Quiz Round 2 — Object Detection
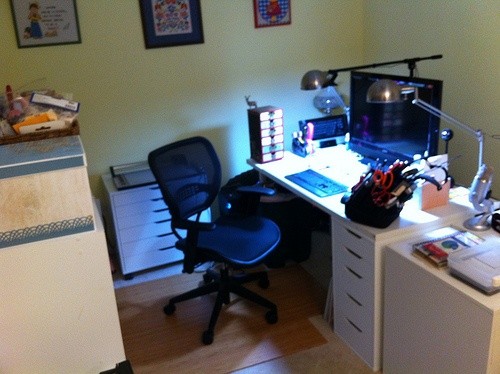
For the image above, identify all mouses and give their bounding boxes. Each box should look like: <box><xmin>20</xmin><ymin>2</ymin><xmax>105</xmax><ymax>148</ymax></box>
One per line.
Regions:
<box><xmin>340</xmin><ymin>192</ymin><xmax>353</xmax><ymax>204</ymax></box>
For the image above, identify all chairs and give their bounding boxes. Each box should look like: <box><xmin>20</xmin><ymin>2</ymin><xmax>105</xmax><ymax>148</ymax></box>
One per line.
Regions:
<box><xmin>150</xmin><ymin>136</ymin><xmax>283</xmax><ymax>345</ymax></box>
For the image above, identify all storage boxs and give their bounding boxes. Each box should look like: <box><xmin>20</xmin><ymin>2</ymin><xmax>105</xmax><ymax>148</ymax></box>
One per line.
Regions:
<box><xmin>0</xmin><ymin>134</ymin><xmax>96</xmax><ymax>249</ymax></box>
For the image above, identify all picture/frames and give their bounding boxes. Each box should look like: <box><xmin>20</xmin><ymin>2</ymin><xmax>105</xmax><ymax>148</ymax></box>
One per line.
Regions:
<box><xmin>253</xmin><ymin>0</ymin><xmax>292</xmax><ymax>28</ymax></box>
<box><xmin>138</xmin><ymin>0</ymin><xmax>204</xmax><ymax>49</ymax></box>
<box><xmin>9</xmin><ymin>0</ymin><xmax>84</xmax><ymax>49</ymax></box>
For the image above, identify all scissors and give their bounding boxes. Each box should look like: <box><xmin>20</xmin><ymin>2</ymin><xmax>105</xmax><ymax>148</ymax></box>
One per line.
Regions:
<box><xmin>373</xmin><ymin>170</ymin><xmax>394</xmax><ymax>205</ymax></box>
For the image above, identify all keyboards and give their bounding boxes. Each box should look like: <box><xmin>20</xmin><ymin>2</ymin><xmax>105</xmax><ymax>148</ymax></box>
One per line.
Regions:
<box><xmin>285</xmin><ymin>169</ymin><xmax>349</xmax><ymax>197</ymax></box>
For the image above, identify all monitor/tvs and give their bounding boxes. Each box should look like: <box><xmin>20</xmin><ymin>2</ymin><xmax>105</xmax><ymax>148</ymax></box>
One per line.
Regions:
<box><xmin>350</xmin><ymin>70</ymin><xmax>444</xmax><ymax>172</ymax></box>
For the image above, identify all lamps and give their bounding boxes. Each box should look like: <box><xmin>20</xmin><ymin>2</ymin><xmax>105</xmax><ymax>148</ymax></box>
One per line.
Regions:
<box><xmin>366</xmin><ymin>80</ymin><xmax>498</xmax><ymax>231</ymax></box>
<box><xmin>300</xmin><ymin>54</ymin><xmax>443</xmax><ymax>91</ymax></box>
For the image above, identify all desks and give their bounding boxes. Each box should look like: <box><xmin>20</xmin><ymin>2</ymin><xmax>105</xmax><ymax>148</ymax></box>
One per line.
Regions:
<box><xmin>0</xmin><ymin>196</ymin><xmax>128</xmax><ymax>374</ymax></box>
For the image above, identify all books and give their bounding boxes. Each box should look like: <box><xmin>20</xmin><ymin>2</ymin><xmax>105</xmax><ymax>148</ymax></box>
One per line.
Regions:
<box><xmin>413</xmin><ymin>231</ymin><xmax>489</xmax><ymax>268</ymax></box>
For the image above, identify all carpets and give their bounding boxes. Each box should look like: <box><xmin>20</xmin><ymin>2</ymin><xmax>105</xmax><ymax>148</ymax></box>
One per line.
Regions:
<box><xmin>232</xmin><ymin>314</ymin><xmax>374</xmax><ymax>374</ymax></box>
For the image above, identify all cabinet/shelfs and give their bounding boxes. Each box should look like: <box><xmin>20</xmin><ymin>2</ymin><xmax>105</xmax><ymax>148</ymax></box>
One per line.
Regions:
<box><xmin>102</xmin><ymin>176</ymin><xmax>212</xmax><ymax>281</ymax></box>
<box><xmin>246</xmin><ymin>144</ymin><xmax>500</xmax><ymax>374</ymax></box>
